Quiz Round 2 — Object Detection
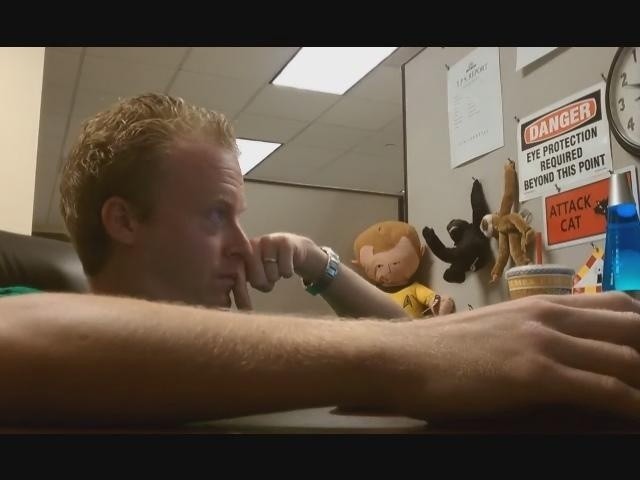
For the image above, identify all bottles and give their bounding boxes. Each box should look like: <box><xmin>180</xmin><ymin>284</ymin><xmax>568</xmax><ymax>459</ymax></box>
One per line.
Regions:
<box><xmin>601</xmin><ymin>172</ymin><xmax>640</xmax><ymax>292</ymax></box>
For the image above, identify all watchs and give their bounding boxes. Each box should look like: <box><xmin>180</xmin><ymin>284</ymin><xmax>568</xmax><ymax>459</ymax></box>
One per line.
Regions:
<box><xmin>301</xmin><ymin>246</ymin><xmax>340</xmax><ymax>296</ymax></box>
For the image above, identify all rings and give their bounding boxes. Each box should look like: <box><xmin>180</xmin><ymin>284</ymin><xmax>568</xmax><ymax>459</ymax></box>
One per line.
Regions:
<box><xmin>263</xmin><ymin>258</ymin><xmax>277</xmax><ymax>263</ymax></box>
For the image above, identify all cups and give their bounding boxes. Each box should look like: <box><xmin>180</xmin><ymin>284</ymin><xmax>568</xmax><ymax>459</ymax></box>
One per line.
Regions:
<box><xmin>505</xmin><ymin>266</ymin><xmax>574</xmax><ymax>300</ymax></box>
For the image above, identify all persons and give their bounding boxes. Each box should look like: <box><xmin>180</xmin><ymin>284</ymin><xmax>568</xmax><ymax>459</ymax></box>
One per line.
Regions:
<box><xmin>0</xmin><ymin>93</ymin><xmax>640</xmax><ymax>429</ymax></box>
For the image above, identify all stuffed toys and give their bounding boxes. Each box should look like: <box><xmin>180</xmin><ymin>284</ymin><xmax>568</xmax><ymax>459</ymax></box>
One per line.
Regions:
<box><xmin>423</xmin><ymin>179</ymin><xmax>489</xmax><ymax>283</ymax></box>
<box><xmin>351</xmin><ymin>220</ymin><xmax>454</xmax><ymax>320</ymax></box>
<box><xmin>480</xmin><ymin>161</ymin><xmax>534</xmax><ymax>286</ymax></box>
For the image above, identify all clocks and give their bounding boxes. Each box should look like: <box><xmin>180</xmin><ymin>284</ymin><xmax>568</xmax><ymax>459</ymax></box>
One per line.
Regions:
<box><xmin>605</xmin><ymin>47</ymin><xmax>640</xmax><ymax>158</ymax></box>
